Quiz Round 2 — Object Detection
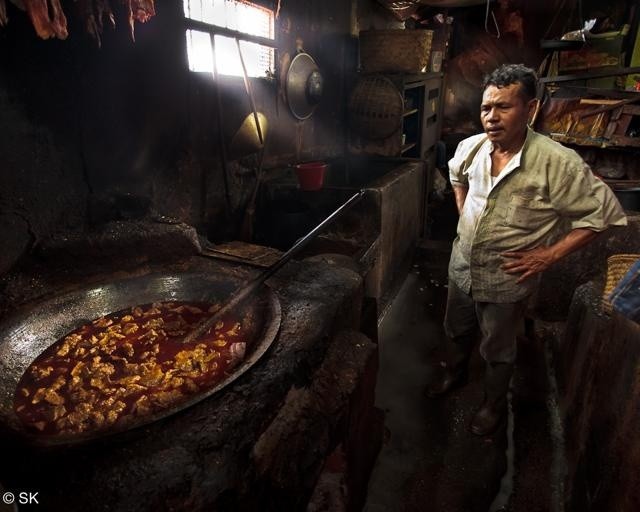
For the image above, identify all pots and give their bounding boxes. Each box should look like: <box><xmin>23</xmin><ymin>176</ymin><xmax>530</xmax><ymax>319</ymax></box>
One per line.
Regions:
<box><xmin>1</xmin><ymin>270</ymin><xmax>284</xmax><ymax>450</ymax></box>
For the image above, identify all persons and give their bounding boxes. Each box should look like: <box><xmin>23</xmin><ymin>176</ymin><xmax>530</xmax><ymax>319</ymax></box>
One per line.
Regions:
<box><xmin>421</xmin><ymin>65</ymin><xmax>629</xmax><ymax>434</ymax></box>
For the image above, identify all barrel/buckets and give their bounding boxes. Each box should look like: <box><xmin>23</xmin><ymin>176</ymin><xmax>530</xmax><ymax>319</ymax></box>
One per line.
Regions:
<box><xmin>294</xmin><ymin>162</ymin><xmax>327</xmax><ymax>191</ymax></box>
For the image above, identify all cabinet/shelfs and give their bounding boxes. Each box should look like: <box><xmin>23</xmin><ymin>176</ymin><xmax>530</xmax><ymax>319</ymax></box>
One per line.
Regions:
<box><xmin>397</xmin><ymin>72</ymin><xmax>442</xmax><ymax>160</ymax></box>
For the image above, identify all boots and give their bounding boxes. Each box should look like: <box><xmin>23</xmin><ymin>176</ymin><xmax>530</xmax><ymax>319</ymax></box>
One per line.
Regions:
<box><xmin>468</xmin><ymin>360</ymin><xmax>514</xmax><ymax>437</ymax></box>
<box><xmin>425</xmin><ymin>340</ymin><xmax>475</xmax><ymax>400</ymax></box>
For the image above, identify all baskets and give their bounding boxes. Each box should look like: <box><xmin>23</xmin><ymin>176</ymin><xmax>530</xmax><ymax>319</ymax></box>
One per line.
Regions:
<box><xmin>358</xmin><ymin>29</ymin><xmax>433</xmax><ymax>74</ymax></box>
<box><xmin>346</xmin><ymin>76</ymin><xmax>405</xmax><ymax>140</ymax></box>
<box><xmin>601</xmin><ymin>254</ymin><xmax>639</xmax><ymax>317</ymax></box>
